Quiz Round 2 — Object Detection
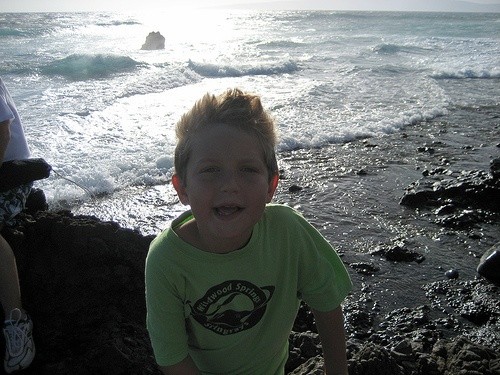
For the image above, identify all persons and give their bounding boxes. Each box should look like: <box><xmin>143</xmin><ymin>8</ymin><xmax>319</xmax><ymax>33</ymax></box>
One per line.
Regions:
<box><xmin>145</xmin><ymin>91</ymin><xmax>353</xmax><ymax>375</ymax></box>
<box><xmin>0</xmin><ymin>78</ymin><xmax>35</xmax><ymax>375</ymax></box>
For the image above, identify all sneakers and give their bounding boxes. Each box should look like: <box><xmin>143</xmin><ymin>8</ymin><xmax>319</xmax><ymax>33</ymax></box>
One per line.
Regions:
<box><xmin>3</xmin><ymin>308</ymin><xmax>38</xmax><ymax>374</ymax></box>
